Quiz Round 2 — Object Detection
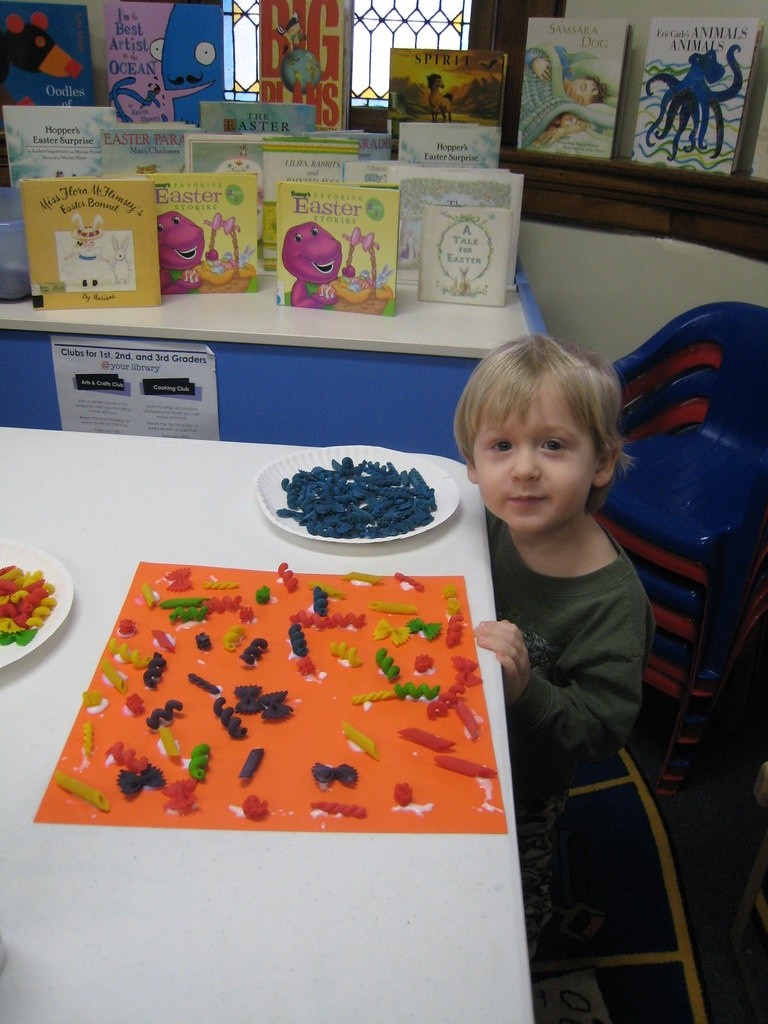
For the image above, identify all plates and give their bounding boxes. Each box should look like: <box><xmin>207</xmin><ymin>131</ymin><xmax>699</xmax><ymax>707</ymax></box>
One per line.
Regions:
<box><xmin>0</xmin><ymin>538</ymin><xmax>75</xmax><ymax>669</ymax></box>
<box><xmin>254</xmin><ymin>443</ymin><xmax>461</xmax><ymax>545</ymax></box>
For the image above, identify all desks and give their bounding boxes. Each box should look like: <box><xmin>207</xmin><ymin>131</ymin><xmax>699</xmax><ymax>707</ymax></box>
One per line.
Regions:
<box><xmin>0</xmin><ymin>427</ymin><xmax>535</xmax><ymax>1024</ymax></box>
<box><xmin>0</xmin><ymin>258</ymin><xmax>548</xmax><ymax>468</ymax></box>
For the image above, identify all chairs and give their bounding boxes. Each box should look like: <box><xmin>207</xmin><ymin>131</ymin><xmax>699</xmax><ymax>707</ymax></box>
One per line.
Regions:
<box><xmin>584</xmin><ymin>303</ymin><xmax>768</xmax><ymax>795</ymax></box>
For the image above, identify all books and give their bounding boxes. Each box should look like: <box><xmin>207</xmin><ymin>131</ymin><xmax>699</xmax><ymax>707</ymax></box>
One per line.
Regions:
<box><xmin>0</xmin><ymin>0</ymin><xmax>524</xmax><ymax>320</ymax></box>
<box><xmin>632</xmin><ymin>16</ymin><xmax>765</xmax><ymax>175</ymax></box>
<box><xmin>750</xmin><ymin>90</ymin><xmax>768</xmax><ymax>183</ymax></box>
<box><xmin>515</xmin><ymin>17</ymin><xmax>635</xmax><ymax>159</ymax></box>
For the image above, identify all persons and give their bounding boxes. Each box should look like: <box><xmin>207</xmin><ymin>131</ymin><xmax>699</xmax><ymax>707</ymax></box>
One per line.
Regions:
<box><xmin>457</xmin><ymin>331</ymin><xmax>654</xmax><ymax>981</ymax></box>
